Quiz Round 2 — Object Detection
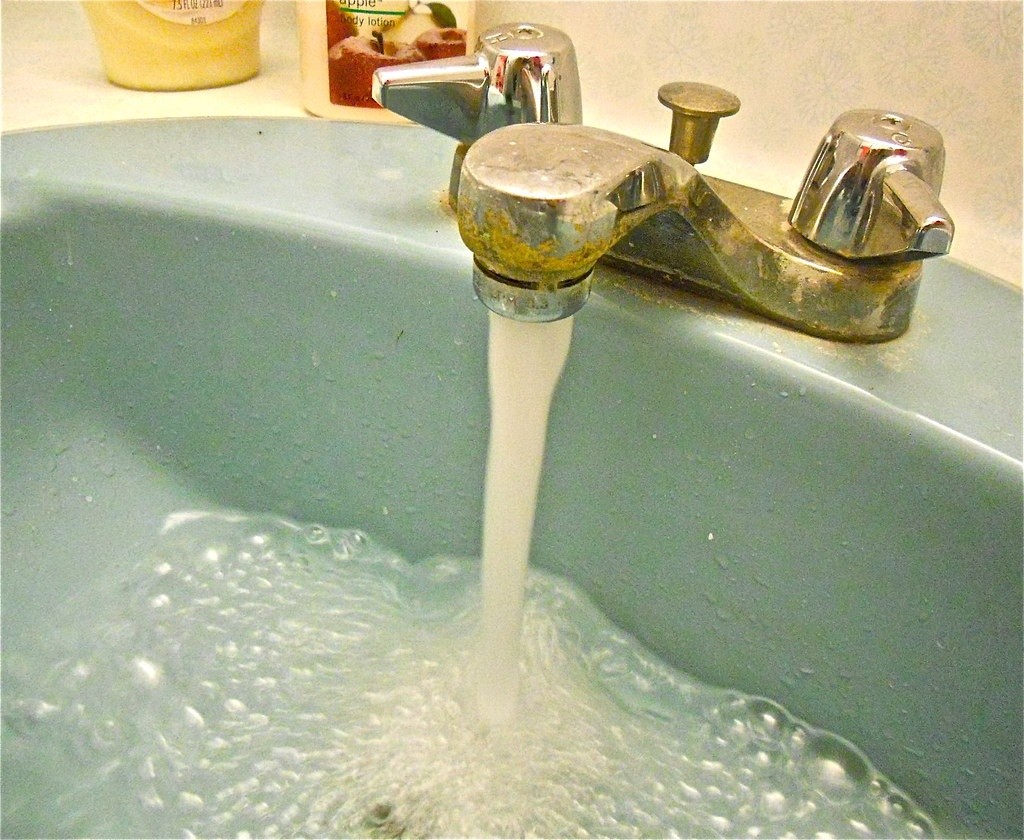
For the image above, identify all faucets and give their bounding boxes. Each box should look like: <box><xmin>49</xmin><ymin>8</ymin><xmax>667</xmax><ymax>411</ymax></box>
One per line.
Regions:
<box><xmin>373</xmin><ymin>23</ymin><xmax>955</xmax><ymax>344</ymax></box>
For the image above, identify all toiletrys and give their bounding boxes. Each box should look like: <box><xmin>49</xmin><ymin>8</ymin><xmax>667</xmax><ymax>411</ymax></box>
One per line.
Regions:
<box><xmin>297</xmin><ymin>0</ymin><xmax>477</xmax><ymax>125</ymax></box>
<box><xmin>82</xmin><ymin>0</ymin><xmax>266</xmax><ymax>92</ymax></box>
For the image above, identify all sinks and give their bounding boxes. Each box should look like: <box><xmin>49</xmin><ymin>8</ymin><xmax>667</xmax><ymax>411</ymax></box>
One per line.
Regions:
<box><xmin>0</xmin><ymin>113</ymin><xmax>1024</xmax><ymax>837</ymax></box>
